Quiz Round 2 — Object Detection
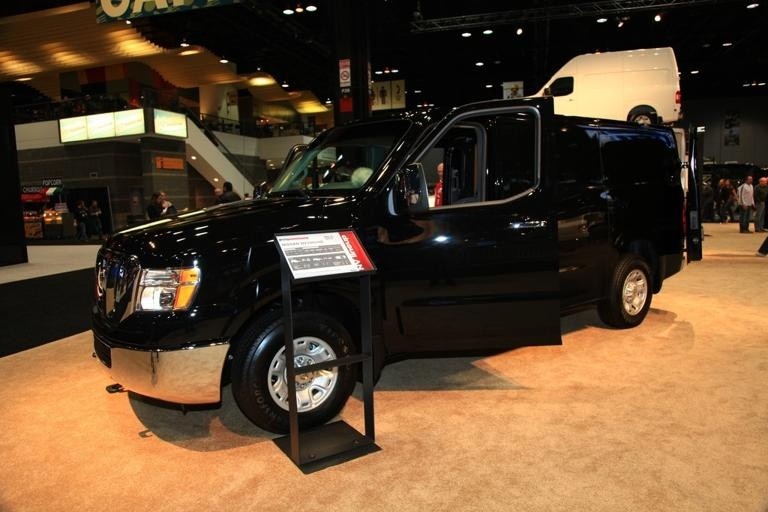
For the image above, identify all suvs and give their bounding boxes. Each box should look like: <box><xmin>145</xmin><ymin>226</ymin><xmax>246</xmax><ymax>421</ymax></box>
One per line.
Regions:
<box><xmin>90</xmin><ymin>96</ymin><xmax>687</xmax><ymax>436</ymax></box>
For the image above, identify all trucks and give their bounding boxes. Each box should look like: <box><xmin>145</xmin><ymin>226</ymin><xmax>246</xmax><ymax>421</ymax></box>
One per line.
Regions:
<box><xmin>525</xmin><ymin>47</ymin><xmax>682</xmax><ymax>125</ymax></box>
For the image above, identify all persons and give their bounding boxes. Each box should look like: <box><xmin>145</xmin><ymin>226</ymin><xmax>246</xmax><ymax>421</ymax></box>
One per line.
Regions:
<box><xmin>756</xmin><ymin>236</ymin><xmax>768</xmax><ymax>255</ymax></box>
<box><xmin>433</xmin><ymin>164</ymin><xmax>444</xmax><ymax>207</ymax></box>
<box><xmin>72</xmin><ymin>201</ymin><xmax>91</xmax><ymax>243</ymax></box>
<box><xmin>703</xmin><ymin>175</ymin><xmax>768</xmax><ymax>235</ymax></box>
<box><xmin>86</xmin><ymin>200</ymin><xmax>107</xmax><ymax>240</ymax></box>
<box><xmin>160</xmin><ymin>192</ymin><xmax>177</xmax><ymax>216</ymax></box>
<box><xmin>216</xmin><ymin>182</ymin><xmax>241</xmax><ymax>205</ymax></box>
<box><xmin>148</xmin><ymin>193</ymin><xmax>169</xmax><ymax>218</ymax></box>
